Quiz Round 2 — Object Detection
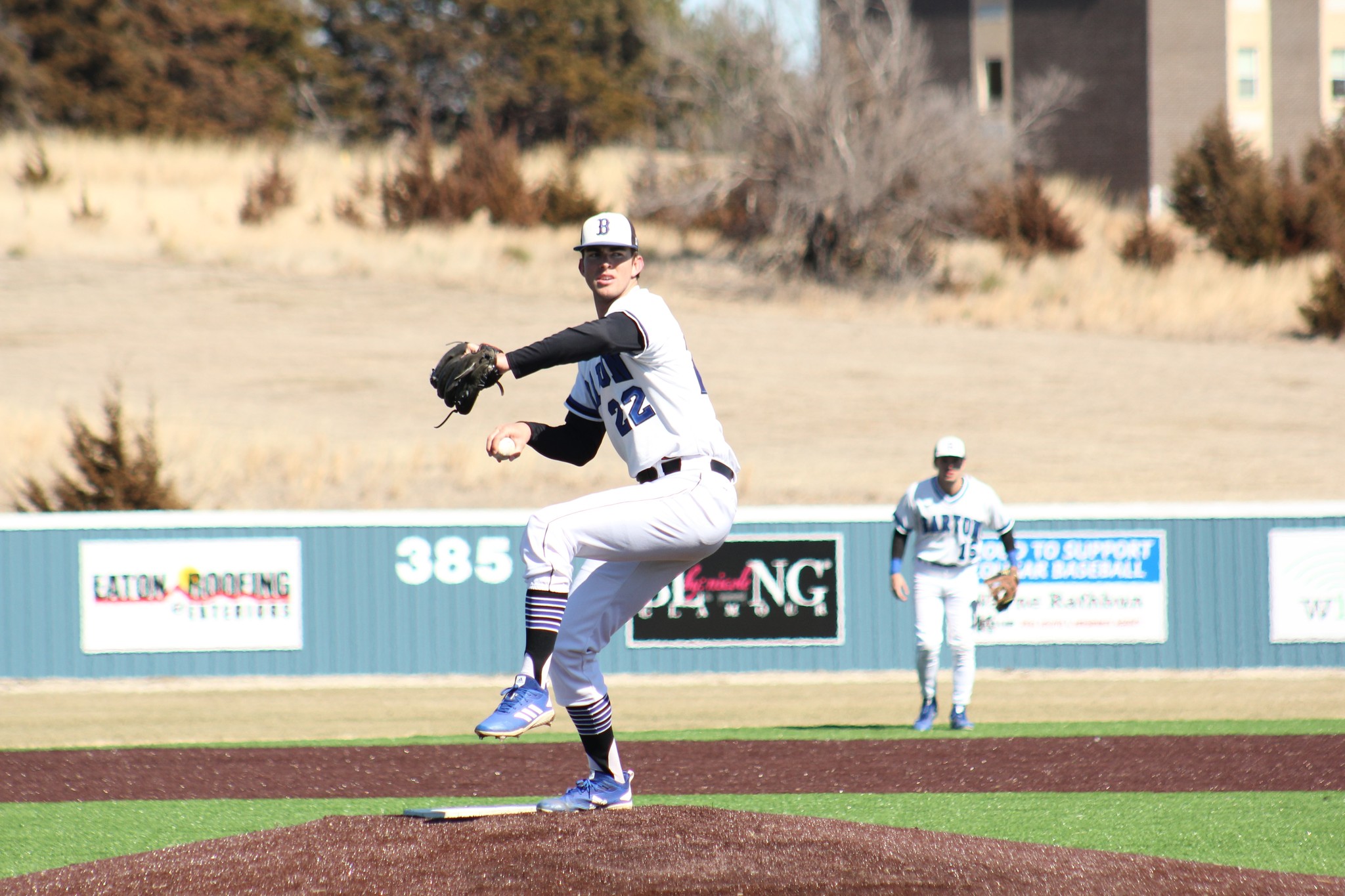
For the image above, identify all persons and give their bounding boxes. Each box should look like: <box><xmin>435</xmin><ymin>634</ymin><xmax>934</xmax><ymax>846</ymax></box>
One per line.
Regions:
<box><xmin>430</xmin><ymin>211</ymin><xmax>741</xmax><ymax>810</ymax></box>
<box><xmin>889</xmin><ymin>436</ymin><xmax>1016</xmax><ymax>734</ymax></box>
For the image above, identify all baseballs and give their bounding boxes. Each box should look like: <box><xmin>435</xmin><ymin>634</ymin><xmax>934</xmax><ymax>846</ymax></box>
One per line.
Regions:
<box><xmin>491</xmin><ymin>437</ymin><xmax>516</xmax><ymax>461</ymax></box>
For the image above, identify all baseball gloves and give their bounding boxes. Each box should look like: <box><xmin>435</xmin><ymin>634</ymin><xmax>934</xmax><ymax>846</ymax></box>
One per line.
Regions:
<box><xmin>430</xmin><ymin>341</ymin><xmax>505</xmax><ymax>415</ymax></box>
<box><xmin>984</xmin><ymin>566</ymin><xmax>1020</xmax><ymax>614</ymax></box>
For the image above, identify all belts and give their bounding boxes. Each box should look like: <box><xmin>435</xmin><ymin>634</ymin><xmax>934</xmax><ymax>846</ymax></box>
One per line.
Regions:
<box><xmin>919</xmin><ymin>558</ymin><xmax>956</xmax><ymax>569</ymax></box>
<box><xmin>637</xmin><ymin>460</ymin><xmax>733</xmax><ymax>484</ymax></box>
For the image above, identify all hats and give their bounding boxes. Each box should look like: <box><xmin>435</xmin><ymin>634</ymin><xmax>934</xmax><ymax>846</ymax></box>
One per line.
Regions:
<box><xmin>573</xmin><ymin>213</ymin><xmax>639</xmax><ymax>249</ymax></box>
<box><xmin>935</xmin><ymin>437</ymin><xmax>965</xmax><ymax>458</ymax></box>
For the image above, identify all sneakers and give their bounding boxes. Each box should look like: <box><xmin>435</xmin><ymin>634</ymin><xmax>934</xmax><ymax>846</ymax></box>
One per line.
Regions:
<box><xmin>949</xmin><ymin>705</ymin><xmax>974</xmax><ymax>731</ymax></box>
<box><xmin>536</xmin><ymin>770</ymin><xmax>635</xmax><ymax>813</ymax></box>
<box><xmin>475</xmin><ymin>674</ymin><xmax>555</xmax><ymax>742</ymax></box>
<box><xmin>913</xmin><ymin>697</ymin><xmax>938</xmax><ymax>731</ymax></box>
